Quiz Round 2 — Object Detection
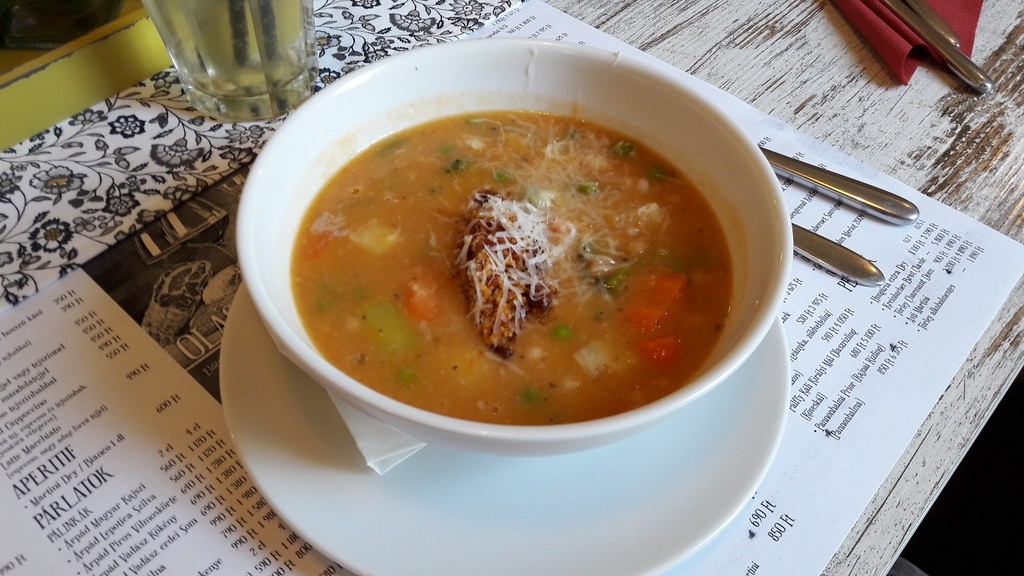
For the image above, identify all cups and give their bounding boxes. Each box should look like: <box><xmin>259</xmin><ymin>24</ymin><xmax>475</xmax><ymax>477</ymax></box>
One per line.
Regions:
<box><xmin>143</xmin><ymin>0</ymin><xmax>317</xmax><ymax>124</ymax></box>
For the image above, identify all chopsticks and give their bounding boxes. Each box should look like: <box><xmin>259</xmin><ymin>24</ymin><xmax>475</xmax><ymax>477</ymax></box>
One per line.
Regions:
<box><xmin>225</xmin><ymin>0</ymin><xmax>290</xmax><ymax>119</ymax></box>
<box><xmin>881</xmin><ymin>1</ymin><xmax>995</xmax><ymax>92</ymax></box>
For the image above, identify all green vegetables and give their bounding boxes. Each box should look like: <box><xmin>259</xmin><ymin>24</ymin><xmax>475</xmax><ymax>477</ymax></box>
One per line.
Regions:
<box><xmin>436</xmin><ymin>119</ymin><xmax>692</xmax><ymax>408</ymax></box>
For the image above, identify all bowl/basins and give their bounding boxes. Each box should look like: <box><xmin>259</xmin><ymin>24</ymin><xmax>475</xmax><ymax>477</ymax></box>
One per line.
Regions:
<box><xmin>236</xmin><ymin>38</ymin><xmax>793</xmax><ymax>453</ymax></box>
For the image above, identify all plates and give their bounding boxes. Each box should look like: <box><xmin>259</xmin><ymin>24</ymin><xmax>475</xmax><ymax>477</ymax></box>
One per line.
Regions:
<box><xmin>219</xmin><ymin>282</ymin><xmax>791</xmax><ymax>576</ymax></box>
